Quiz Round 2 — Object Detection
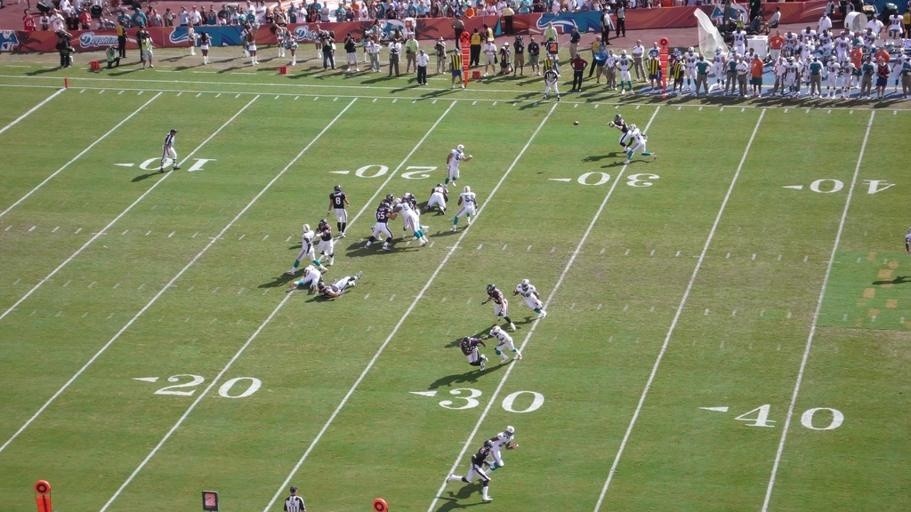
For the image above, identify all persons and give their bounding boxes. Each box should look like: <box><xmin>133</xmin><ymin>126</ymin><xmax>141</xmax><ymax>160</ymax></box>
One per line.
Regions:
<box><xmin>445</xmin><ymin>144</ymin><xmax>473</xmax><ymax>187</ymax></box>
<box><xmin>445</xmin><ymin>438</ymin><xmax>495</xmax><ymax>502</ymax></box>
<box><xmin>513</xmin><ymin>278</ymin><xmax>548</xmax><ymax>318</ymax></box>
<box><xmin>481</xmin><ymin>323</ymin><xmax>524</xmax><ymax>363</ymax></box>
<box><xmin>483</xmin><ymin>423</ymin><xmax>520</xmax><ymax>476</ymax></box>
<box><xmin>0</xmin><ymin>0</ymin><xmax>911</xmax><ymax>107</ymax></box>
<box><xmin>160</xmin><ymin>128</ymin><xmax>181</xmax><ymax>174</ymax></box>
<box><xmin>284</xmin><ymin>183</ymin><xmax>480</xmax><ymax>303</ymax></box>
<box><xmin>461</xmin><ymin>335</ymin><xmax>488</xmax><ymax>376</ymax></box>
<box><xmin>622</xmin><ymin>123</ymin><xmax>658</xmax><ymax>166</ymax></box>
<box><xmin>904</xmin><ymin>227</ymin><xmax>911</xmax><ymax>257</ymax></box>
<box><xmin>481</xmin><ymin>282</ymin><xmax>518</xmax><ymax>332</ymax></box>
<box><xmin>282</xmin><ymin>485</ymin><xmax>306</xmax><ymax>512</ymax></box>
<box><xmin>610</xmin><ymin>113</ymin><xmax>648</xmax><ymax>154</ymax></box>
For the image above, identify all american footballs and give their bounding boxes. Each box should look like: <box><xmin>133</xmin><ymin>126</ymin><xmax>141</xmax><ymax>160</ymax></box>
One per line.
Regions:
<box><xmin>508</xmin><ymin>440</ymin><xmax>517</xmax><ymax>449</ymax></box>
<box><xmin>573</xmin><ymin>122</ymin><xmax>580</xmax><ymax>125</ymax></box>
